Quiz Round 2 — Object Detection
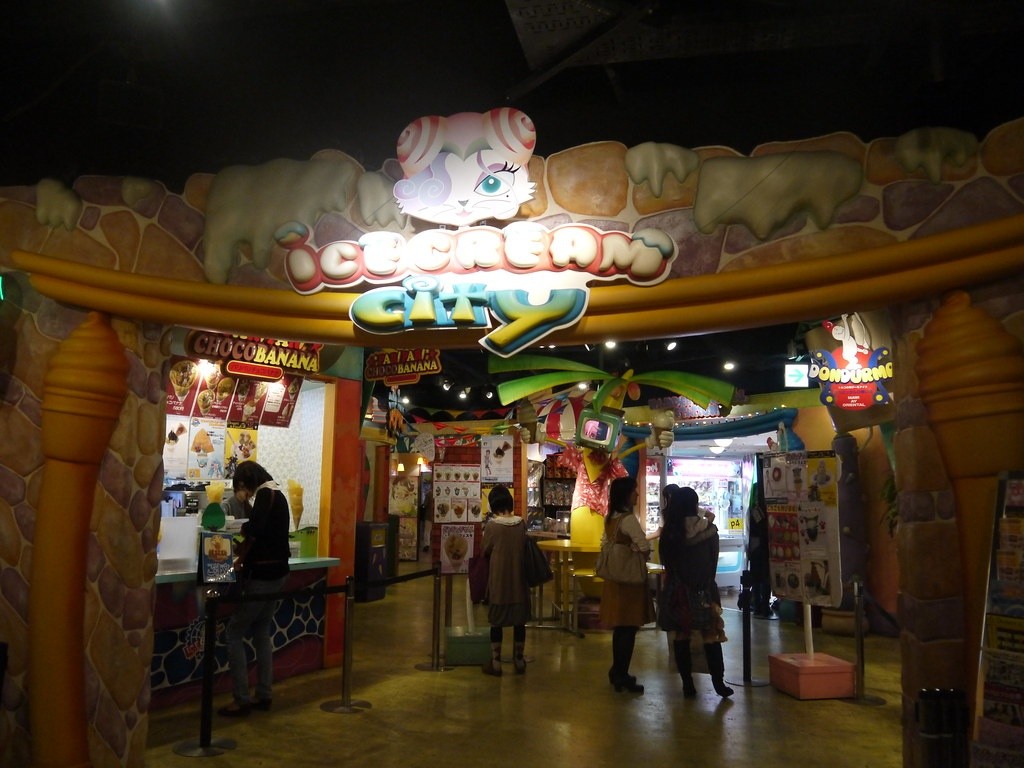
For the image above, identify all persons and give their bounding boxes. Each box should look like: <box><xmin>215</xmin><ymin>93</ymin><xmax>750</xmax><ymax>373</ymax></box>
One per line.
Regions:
<box><xmin>600</xmin><ymin>477</ymin><xmax>652</xmax><ymax>695</ymax></box>
<box><xmin>480</xmin><ymin>486</ymin><xmax>531</xmax><ymax>677</ymax></box>
<box><xmin>214</xmin><ymin>461</ymin><xmax>289</xmax><ymax>719</ymax></box>
<box><xmin>659</xmin><ymin>485</ymin><xmax>734</xmax><ymax>697</ymax></box>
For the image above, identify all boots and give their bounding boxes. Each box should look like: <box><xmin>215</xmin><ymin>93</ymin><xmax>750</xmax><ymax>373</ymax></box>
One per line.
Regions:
<box><xmin>672</xmin><ymin>639</ymin><xmax>696</xmax><ymax>699</ymax></box>
<box><xmin>705</xmin><ymin>641</ymin><xmax>733</xmax><ymax>696</ymax></box>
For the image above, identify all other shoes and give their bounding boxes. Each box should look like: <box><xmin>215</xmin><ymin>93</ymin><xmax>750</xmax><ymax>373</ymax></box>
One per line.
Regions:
<box><xmin>514</xmin><ymin>660</ymin><xmax>526</xmax><ymax>673</ymax></box>
<box><xmin>483</xmin><ymin>658</ymin><xmax>503</xmax><ymax>676</ymax></box>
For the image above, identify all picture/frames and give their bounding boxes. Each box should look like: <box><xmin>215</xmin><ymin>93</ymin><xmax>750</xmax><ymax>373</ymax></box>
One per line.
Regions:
<box><xmin>389</xmin><ymin>474</ymin><xmax>421</xmax><ymax>516</ymax></box>
<box><xmin>395</xmin><ymin>516</ymin><xmax>419</xmax><ymax>561</ymax></box>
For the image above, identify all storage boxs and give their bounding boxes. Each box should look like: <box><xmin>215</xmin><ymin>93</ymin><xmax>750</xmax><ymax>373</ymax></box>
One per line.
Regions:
<box><xmin>156</xmin><ymin>516</ymin><xmax>197</xmax><ymax>573</ymax></box>
<box><xmin>289</xmin><ymin>527</ymin><xmax>316</xmax><ymax>557</ymax></box>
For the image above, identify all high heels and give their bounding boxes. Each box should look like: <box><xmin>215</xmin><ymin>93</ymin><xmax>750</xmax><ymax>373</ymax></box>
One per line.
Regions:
<box><xmin>615</xmin><ymin>674</ymin><xmax>644</xmax><ymax>694</ymax></box>
<box><xmin>221</xmin><ymin>704</ymin><xmax>250</xmax><ymax>716</ymax></box>
<box><xmin>250</xmin><ymin>697</ymin><xmax>273</xmax><ymax>712</ymax></box>
<box><xmin>608</xmin><ymin>669</ymin><xmax>636</xmax><ymax>686</ymax></box>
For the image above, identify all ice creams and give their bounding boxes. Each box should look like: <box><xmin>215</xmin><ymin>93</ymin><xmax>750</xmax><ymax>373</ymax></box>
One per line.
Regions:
<box><xmin>169</xmin><ymin>358</ymin><xmax>301</xmax><ymax>463</ymax></box>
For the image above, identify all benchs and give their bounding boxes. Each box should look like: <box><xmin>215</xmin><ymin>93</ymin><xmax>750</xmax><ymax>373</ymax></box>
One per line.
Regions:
<box><xmin>570</xmin><ymin>562</ymin><xmax>665</xmax><ymax>638</ymax></box>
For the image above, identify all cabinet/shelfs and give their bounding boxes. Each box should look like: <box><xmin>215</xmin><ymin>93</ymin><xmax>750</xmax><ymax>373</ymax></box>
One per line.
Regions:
<box><xmin>539</xmin><ymin>476</ymin><xmax>576</xmax><ymax>518</ymax></box>
<box><xmin>355</xmin><ymin>521</ymin><xmax>387</xmax><ymax>601</ymax></box>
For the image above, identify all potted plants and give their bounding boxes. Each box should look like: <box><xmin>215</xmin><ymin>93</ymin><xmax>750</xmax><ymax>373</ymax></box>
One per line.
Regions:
<box><xmin>821</xmin><ymin>593</ymin><xmax>870</xmax><ymax>635</ymax></box>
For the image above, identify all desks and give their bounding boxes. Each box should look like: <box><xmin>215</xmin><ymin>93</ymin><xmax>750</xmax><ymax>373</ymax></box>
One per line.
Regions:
<box><xmin>537</xmin><ymin>539</ymin><xmax>603</xmax><ymax>633</ymax></box>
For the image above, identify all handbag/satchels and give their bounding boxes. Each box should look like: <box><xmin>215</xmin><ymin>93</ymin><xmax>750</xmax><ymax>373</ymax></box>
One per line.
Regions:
<box><xmin>704</xmin><ymin>584</ymin><xmax>723</xmax><ymax>615</ymax></box>
<box><xmin>248</xmin><ymin>559</ymin><xmax>289</xmax><ymax>601</ymax></box>
<box><xmin>466</xmin><ymin>556</ymin><xmax>491</xmax><ymax>603</ymax></box>
<box><xmin>523</xmin><ymin>537</ymin><xmax>554</xmax><ymax>588</ymax></box>
<box><xmin>597</xmin><ymin>512</ymin><xmax>649</xmax><ymax>586</ymax></box>
